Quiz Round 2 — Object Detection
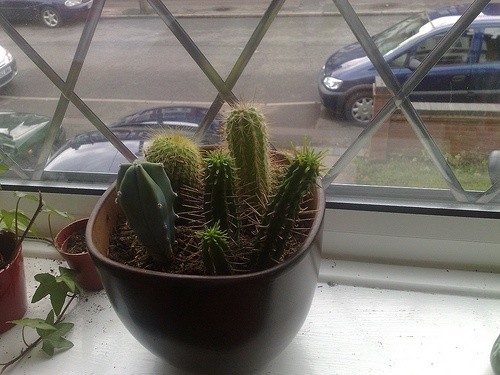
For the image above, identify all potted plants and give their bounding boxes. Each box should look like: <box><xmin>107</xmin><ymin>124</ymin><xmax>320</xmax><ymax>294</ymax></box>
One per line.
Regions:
<box><xmin>85</xmin><ymin>96</ymin><xmax>331</xmax><ymax>375</ymax></box>
<box><xmin>0</xmin><ymin>160</ymin><xmax>87</xmax><ymax>375</ymax></box>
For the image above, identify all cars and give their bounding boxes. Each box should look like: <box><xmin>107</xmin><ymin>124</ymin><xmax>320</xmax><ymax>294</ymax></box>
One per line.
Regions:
<box><xmin>0</xmin><ymin>0</ymin><xmax>94</xmax><ymax>26</ymax></box>
<box><xmin>319</xmin><ymin>3</ymin><xmax>500</xmax><ymax>127</ymax></box>
<box><xmin>0</xmin><ymin>105</ymin><xmax>228</xmax><ymax>183</ymax></box>
<box><xmin>0</xmin><ymin>45</ymin><xmax>19</xmax><ymax>91</ymax></box>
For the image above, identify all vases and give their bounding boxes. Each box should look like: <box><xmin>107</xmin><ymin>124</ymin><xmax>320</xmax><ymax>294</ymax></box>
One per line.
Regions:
<box><xmin>54</xmin><ymin>217</ymin><xmax>103</xmax><ymax>292</ymax></box>
